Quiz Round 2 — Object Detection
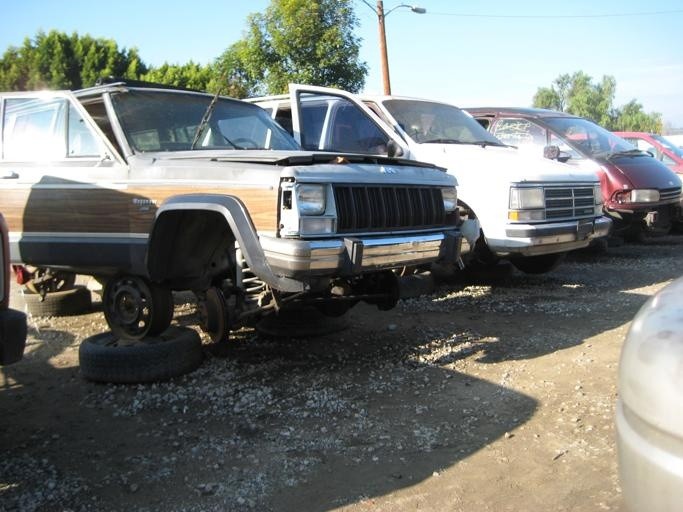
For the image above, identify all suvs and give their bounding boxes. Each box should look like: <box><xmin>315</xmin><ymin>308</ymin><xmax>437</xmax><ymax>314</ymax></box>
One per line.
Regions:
<box><xmin>239</xmin><ymin>94</ymin><xmax>613</xmax><ymax>275</ymax></box>
<box><xmin>0</xmin><ymin>82</ymin><xmax>461</xmax><ymax>341</ymax></box>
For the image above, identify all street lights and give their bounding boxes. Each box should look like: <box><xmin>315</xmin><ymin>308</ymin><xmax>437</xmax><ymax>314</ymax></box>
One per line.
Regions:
<box><xmin>378</xmin><ymin>4</ymin><xmax>426</xmax><ymax>96</ymax></box>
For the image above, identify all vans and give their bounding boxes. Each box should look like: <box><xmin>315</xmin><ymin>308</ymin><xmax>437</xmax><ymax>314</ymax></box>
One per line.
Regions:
<box><xmin>460</xmin><ymin>106</ymin><xmax>683</xmax><ymax>246</ymax></box>
